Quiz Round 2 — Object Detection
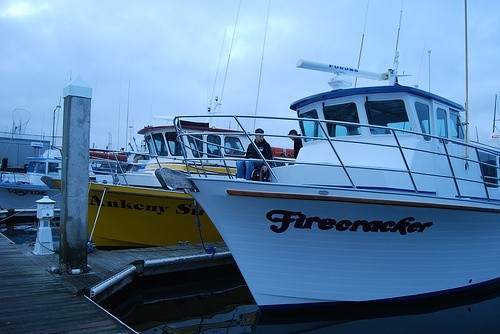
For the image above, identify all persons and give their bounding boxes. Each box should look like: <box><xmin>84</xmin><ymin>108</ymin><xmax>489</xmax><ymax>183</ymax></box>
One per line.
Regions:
<box><xmin>246</xmin><ymin>128</ymin><xmax>272</xmax><ymax>180</ymax></box>
<box><xmin>288</xmin><ymin>129</ymin><xmax>303</xmax><ymax>159</ymax></box>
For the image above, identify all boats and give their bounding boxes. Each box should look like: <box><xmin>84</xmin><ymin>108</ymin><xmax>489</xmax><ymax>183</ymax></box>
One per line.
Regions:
<box><xmin>40</xmin><ymin>0</ymin><xmax>295</xmax><ymax>250</ymax></box>
<box><xmin>0</xmin><ymin>76</ymin><xmax>148</xmax><ymax>211</ymax></box>
<box><xmin>155</xmin><ymin>0</ymin><xmax>500</xmax><ymax>311</ymax></box>
